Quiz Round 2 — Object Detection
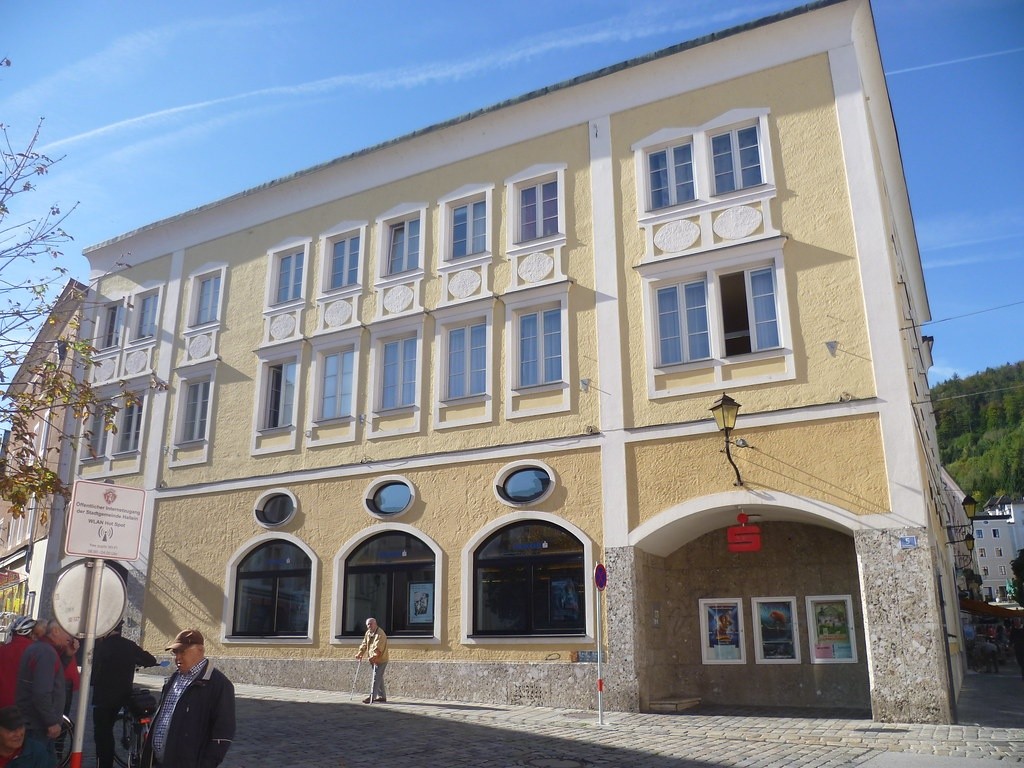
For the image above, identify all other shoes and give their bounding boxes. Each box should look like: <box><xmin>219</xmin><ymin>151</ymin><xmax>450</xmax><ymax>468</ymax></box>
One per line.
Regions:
<box><xmin>362</xmin><ymin>696</ymin><xmax>387</xmax><ymax>703</ymax></box>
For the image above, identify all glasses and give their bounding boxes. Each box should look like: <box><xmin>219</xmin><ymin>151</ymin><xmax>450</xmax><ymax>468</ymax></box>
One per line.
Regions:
<box><xmin>170</xmin><ymin>649</ymin><xmax>187</xmax><ymax>656</ymax></box>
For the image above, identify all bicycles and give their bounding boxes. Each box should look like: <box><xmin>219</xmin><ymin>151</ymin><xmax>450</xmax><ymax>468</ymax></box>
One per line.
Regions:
<box><xmin>49</xmin><ymin>709</ymin><xmax>75</xmax><ymax>767</ymax></box>
<box><xmin>110</xmin><ymin>658</ymin><xmax>171</xmax><ymax>768</ymax></box>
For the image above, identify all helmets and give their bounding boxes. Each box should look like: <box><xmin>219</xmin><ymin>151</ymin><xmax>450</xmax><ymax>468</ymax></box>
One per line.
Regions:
<box><xmin>10</xmin><ymin>615</ymin><xmax>36</xmax><ymax>636</ymax></box>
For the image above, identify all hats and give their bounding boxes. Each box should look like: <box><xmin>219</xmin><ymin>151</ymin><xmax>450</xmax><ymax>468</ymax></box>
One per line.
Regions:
<box><xmin>164</xmin><ymin>630</ymin><xmax>204</xmax><ymax>652</ymax></box>
<box><xmin>0</xmin><ymin>707</ymin><xmax>29</xmax><ymax>729</ymax></box>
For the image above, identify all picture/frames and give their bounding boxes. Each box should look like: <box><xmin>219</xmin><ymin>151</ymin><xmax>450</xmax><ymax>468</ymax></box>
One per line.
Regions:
<box><xmin>750</xmin><ymin>596</ymin><xmax>801</xmax><ymax>665</ymax></box>
<box><xmin>407</xmin><ymin>581</ymin><xmax>435</xmax><ymax>628</ymax></box>
<box><xmin>699</xmin><ymin>598</ymin><xmax>746</xmax><ymax>664</ymax></box>
<box><xmin>804</xmin><ymin>595</ymin><xmax>859</xmax><ymax>664</ymax></box>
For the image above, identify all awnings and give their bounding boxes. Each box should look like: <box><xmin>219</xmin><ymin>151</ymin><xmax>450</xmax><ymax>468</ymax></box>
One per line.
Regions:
<box><xmin>960</xmin><ymin>597</ymin><xmax>1023</xmax><ymax>618</ymax></box>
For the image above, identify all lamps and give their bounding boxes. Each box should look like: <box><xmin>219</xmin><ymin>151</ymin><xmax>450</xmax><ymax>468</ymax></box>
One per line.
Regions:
<box><xmin>708</xmin><ymin>391</ymin><xmax>744</xmax><ymax>487</ymax></box>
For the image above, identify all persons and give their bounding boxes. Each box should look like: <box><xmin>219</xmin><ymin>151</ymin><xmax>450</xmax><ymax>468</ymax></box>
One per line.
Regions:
<box><xmin>415</xmin><ymin>592</ymin><xmax>428</xmax><ymax>613</ymax></box>
<box><xmin>356</xmin><ymin>618</ymin><xmax>389</xmax><ymax>703</ymax></box>
<box><xmin>973</xmin><ymin>642</ymin><xmax>998</xmax><ymax>674</ymax></box>
<box><xmin>1</xmin><ymin>615</ymin><xmax>81</xmax><ymax>758</ymax></box>
<box><xmin>1009</xmin><ymin>617</ymin><xmax>1024</xmax><ymax>683</ymax></box>
<box><xmin>144</xmin><ymin>629</ymin><xmax>236</xmax><ymax>768</ymax></box>
<box><xmin>1</xmin><ymin>705</ymin><xmax>61</xmax><ymax>768</ymax></box>
<box><xmin>89</xmin><ymin>621</ymin><xmax>158</xmax><ymax>768</ymax></box>
